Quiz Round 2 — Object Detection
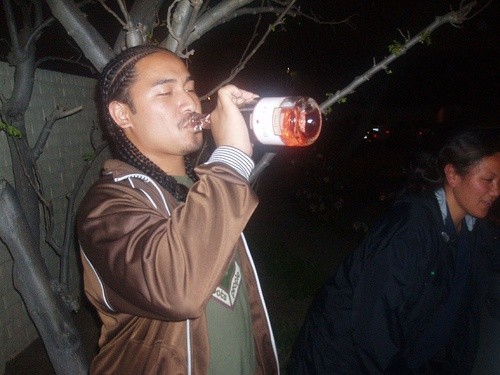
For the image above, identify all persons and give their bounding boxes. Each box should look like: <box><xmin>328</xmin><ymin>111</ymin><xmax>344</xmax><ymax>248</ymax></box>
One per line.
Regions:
<box><xmin>75</xmin><ymin>45</ymin><xmax>280</xmax><ymax>375</ymax></box>
<box><xmin>284</xmin><ymin>132</ymin><xmax>500</xmax><ymax>375</ymax></box>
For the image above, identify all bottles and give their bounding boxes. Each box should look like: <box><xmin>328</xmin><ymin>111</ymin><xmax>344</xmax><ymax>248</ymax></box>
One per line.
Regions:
<box><xmin>197</xmin><ymin>95</ymin><xmax>323</xmax><ymax>147</ymax></box>
